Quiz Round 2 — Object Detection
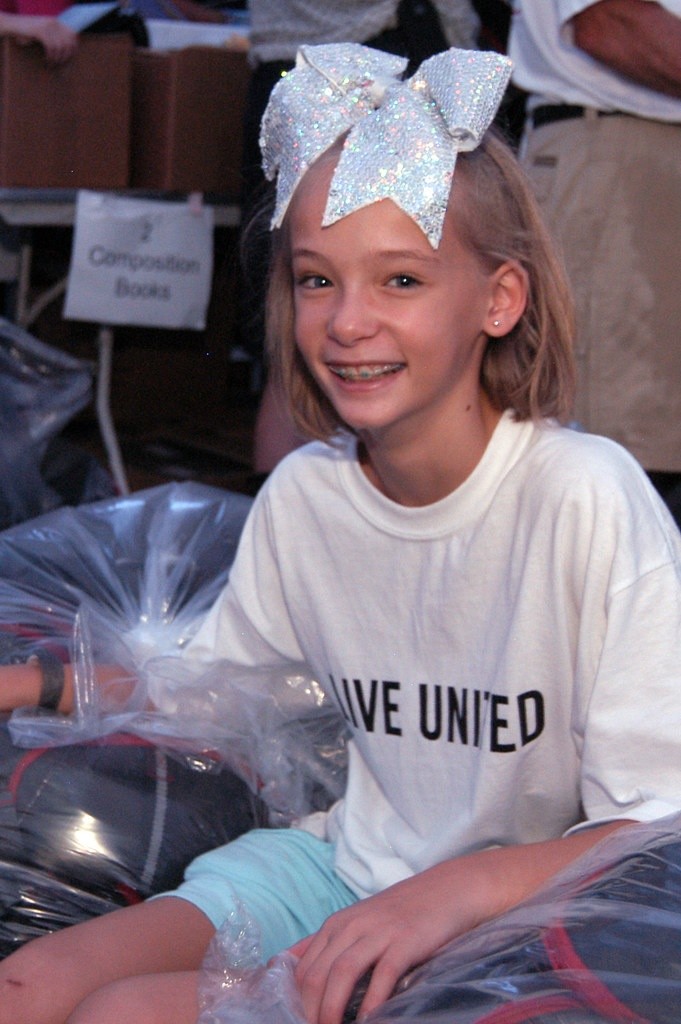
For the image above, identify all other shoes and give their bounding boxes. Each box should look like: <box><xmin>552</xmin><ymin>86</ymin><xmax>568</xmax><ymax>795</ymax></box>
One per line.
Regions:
<box><xmin>229</xmin><ymin>355</ymin><xmax>263</xmax><ymax>408</ymax></box>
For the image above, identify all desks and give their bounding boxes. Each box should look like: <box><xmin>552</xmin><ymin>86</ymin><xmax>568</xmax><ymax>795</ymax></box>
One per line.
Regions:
<box><xmin>1</xmin><ymin>204</ymin><xmax>239</xmax><ymax>503</ymax></box>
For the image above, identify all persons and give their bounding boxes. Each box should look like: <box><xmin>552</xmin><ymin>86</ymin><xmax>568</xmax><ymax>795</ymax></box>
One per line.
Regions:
<box><xmin>0</xmin><ymin>44</ymin><xmax>681</xmax><ymax>1024</ymax></box>
<box><xmin>0</xmin><ymin>0</ymin><xmax>76</xmax><ymax>64</ymax></box>
<box><xmin>230</xmin><ymin>0</ymin><xmax>479</xmax><ymax>400</ymax></box>
<box><xmin>508</xmin><ymin>0</ymin><xmax>680</xmax><ymax>530</ymax></box>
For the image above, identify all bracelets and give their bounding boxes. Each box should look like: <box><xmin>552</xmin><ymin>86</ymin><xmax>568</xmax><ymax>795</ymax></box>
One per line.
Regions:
<box><xmin>25</xmin><ymin>648</ymin><xmax>65</xmax><ymax>714</ymax></box>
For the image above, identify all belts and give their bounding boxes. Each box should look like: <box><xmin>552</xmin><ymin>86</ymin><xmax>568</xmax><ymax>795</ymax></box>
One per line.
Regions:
<box><xmin>531</xmin><ymin>103</ymin><xmax>624</xmax><ymax>128</ymax></box>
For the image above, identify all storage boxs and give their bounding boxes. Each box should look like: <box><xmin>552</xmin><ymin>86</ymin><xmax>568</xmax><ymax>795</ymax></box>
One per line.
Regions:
<box><xmin>130</xmin><ymin>46</ymin><xmax>248</xmax><ymax>200</ymax></box>
<box><xmin>0</xmin><ymin>36</ymin><xmax>135</xmax><ymax>190</ymax></box>
